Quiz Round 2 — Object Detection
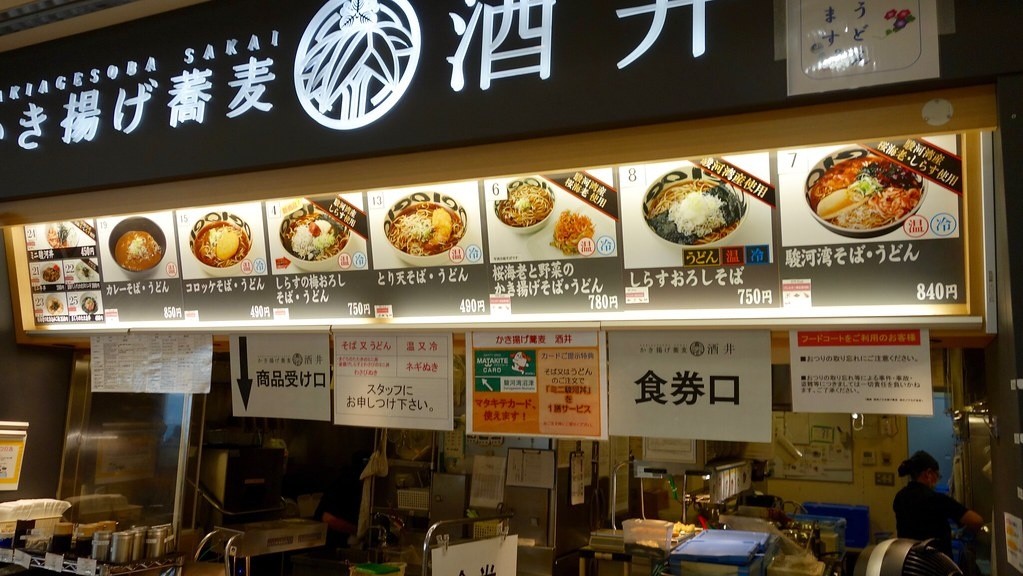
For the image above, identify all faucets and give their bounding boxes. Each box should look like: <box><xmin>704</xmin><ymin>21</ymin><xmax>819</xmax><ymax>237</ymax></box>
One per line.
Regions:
<box><xmin>356</xmin><ymin>525</ymin><xmax>389</xmax><ymax>546</ymax></box>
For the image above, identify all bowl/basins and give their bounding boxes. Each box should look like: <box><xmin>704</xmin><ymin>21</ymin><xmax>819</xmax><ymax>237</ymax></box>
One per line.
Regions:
<box><xmin>39</xmin><ymin>263</ymin><xmax>62</xmax><ymax>283</ymax></box>
<box><xmin>45</xmin><ymin>294</ymin><xmax>64</xmax><ymax>314</ymax></box>
<box><xmin>187</xmin><ymin>211</ymin><xmax>254</xmax><ymax>277</ymax></box>
<box><xmin>277</xmin><ymin>202</ymin><xmax>352</xmax><ymax>273</ymax></box>
<box><xmin>493</xmin><ymin>176</ymin><xmax>556</xmax><ymax>235</ymax></box>
<box><xmin>74</xmin><ymin>261</ymin><xmax>95</xmax><ymax>281</ymax></box>
<box><xmin>79</xmin><ymin>292</ymin><xmax>98</xmax><ymax>314</ymax></box>
<box><xmin>803</xmin><ymin>144</ymin><xmax>929</xmax><ymax>239</ymax></box>
<box><xmin>641</xmin><ymin>166</ymin><xmax>750</xmax><ymax>249</ymax></box>
<box><xmin>383</xmin><ymin>190</ymin><xmax>469</xmax><ymax>267</ymax></box>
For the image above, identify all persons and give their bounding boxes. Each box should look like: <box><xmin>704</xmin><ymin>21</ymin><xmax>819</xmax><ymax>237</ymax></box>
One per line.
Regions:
<box><xmin>892</xmin><ymin>450</ymin><xmax>984</xmax><ymax>565</ymax></box>
<box><xmin>226</xmin><ymin>436</ymin><xmax>305</xmax><ymax>524</ymax></box>
<box><xmin>312</xmin><ymin>465</ymin><xmax>385</xmax><ymax>561</ymax></box>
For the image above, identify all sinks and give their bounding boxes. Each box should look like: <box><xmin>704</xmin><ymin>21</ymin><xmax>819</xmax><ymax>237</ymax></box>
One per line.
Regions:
<box><xmin>290</xmin><ymin>545</ymin><xmax>400</xmax><ymax>576</ymax></box>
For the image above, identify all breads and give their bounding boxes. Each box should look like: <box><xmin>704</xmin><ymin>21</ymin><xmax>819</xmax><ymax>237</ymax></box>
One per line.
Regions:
<box><xmin>631</xmin><ymin>521</ymin><xmax>696</xmax><ymax>537</ymax></box>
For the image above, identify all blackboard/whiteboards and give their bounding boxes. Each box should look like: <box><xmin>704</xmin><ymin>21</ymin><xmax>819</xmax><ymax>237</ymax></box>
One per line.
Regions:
<box><xmin>772</xmin><ymin>411</ymin><xmax>853</xmax><ymax>483</ymax></box>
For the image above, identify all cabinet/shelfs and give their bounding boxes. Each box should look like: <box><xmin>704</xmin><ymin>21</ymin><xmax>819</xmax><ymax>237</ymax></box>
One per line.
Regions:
<box><xmin>367</xmin><ymin>428</ymin><xmax>440</xmax><ymax>565</ymax></box>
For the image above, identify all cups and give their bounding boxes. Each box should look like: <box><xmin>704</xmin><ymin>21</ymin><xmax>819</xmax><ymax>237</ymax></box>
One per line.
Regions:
<box><xmin>92</xmin><ymin>523</ymin><xmax>175</xmax><ymax>564</ymax></box>
<box><xmin>49</xmin><ymin>534</ymin><xmax>73</xmax><ymax>555</ymax></box>
<box><xmin>75</xmin><ymin>537</ymin><xmax>93</xmax><ymax>558</ymax></box>
<box><xmin>108</xmin><ymin>216</ymin><xmax>167</xmax><ymax>277</ymax></box>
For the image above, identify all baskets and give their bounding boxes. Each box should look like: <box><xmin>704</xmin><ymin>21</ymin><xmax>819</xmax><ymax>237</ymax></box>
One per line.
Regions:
<box><xmin>397</xmin><ymin>489</ymin><xmax>430</xmax><ymax>511</ymax></box>
<box><xmin>473</xmin><ymin>521</ymin><xmax>504</xmax><ymax>539</ymax></box>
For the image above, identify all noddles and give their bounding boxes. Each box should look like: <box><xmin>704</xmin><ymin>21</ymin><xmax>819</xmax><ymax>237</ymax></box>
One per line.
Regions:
<box><xmin>285</xmin><ymin>213</ymin><xmax>348</xmax><ymax>262</ymax></box>
<box><xmin>194</xmin><ymin>221</ymin><xmax>248</xmax><ymax>268</ymax></box>
<box><xmin>496</xmin><ymin>186</ymin><xmax>553</xmax><ymax>227</ymax></box>
<box><xmin>808</xmin><ymin>157</ymin><xmax>922</xmax><ymax>231</ymax></box>
<box><xmin>386</xmin><ymin>202</ymin><xmax>464</xmax><ymax>256</ymax></box>
<box><xmin>647</xmin><ymin>179</ymin><xmax>740</xmax><ymax>245</ymax></box>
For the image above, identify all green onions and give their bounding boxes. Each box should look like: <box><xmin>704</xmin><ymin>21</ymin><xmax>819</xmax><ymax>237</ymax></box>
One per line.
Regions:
<box><xmin>848</xmin><ymin>173</ymin><xmax>884</xmax><ymax>200</ymax></box>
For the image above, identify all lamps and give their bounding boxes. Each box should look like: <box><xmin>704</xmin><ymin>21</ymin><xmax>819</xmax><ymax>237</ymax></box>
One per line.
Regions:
<box><xmin>682</xmin><ymin>469</ymin><xmax>712</xmax><ymax>523</ymax></box>
<box><xmin>639</xmin><ymin>468</ymin><xmax>667</xmax><ymax>519</ymax></box>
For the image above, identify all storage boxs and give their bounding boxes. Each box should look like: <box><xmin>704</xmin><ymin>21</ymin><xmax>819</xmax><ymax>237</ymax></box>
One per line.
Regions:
<box><xmin>622</xmin><ymin>518</ymin><xmax>674</xmax><ymax>558</ymax></box>
<box><xmin>669</xmin><ymin>529</ymin><xmax>772</xmax><ymax>576</ymax></box>
<box><xmin>785</xmin><ymin>502</ymin><xmax>872</xmax><ymax>554</ymax></box>
